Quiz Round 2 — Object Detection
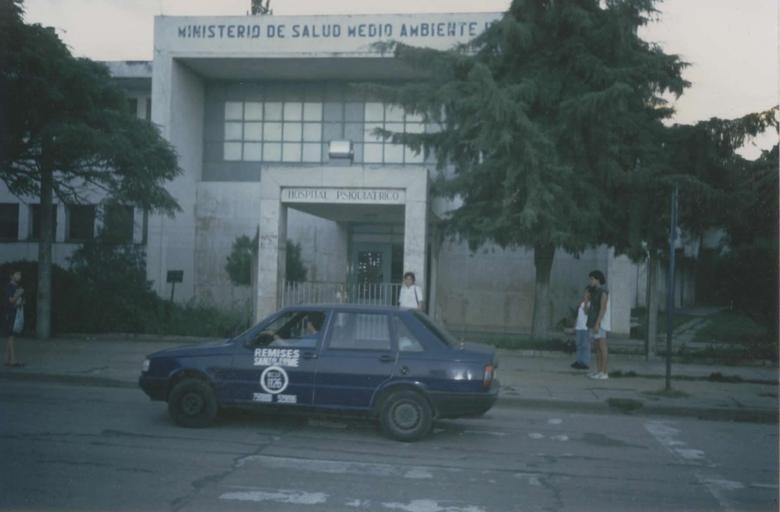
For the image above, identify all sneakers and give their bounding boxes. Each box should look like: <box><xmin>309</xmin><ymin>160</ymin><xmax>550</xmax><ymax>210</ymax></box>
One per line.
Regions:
<box><xmin>571</xmin><ymin>361</ymin><xmax>589</xmax><ymax>369</ymax></box>
<box><xmin>587</xmin><ymin>370</ymin><xmax>608</xmax><ymax>379</ymax></box>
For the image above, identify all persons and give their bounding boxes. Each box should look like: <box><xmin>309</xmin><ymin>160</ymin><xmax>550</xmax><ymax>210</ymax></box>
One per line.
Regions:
<box><xmin>583</xmin><ymin>271</ymin><xmax>610</xmax><ymax>381</ymax></box>
<box><xmin>2</xmin><ymin>268</ymin><xmax>27</xmax><ymax>368</ymax></box>
<box><xmin>571</xmin><ymin>287</ymin><xmax>592</xmax><ymax>371</ymax></box>
<box><xmin>397</xmin><ymin>273</ymin><xmax>423</xmax><ymax>309</ymax></box>
<box><xmin>260</xmin><ymin>312</ymin><xmax>325</xmax><ymax>348</ymax></box>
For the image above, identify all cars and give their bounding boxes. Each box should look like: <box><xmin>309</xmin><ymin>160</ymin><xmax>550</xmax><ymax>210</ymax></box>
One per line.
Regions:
<box><xmin>140</xmin><ymin>301</ymin><xmax>501</xmax><ymax>441</ymax></box>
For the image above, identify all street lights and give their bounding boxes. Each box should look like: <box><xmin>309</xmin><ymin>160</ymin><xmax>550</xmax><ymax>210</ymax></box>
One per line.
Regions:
<box><xmin>656</xmin><ymin>161</ymin><xmax>691</xmax><ymax>395</ymax></box>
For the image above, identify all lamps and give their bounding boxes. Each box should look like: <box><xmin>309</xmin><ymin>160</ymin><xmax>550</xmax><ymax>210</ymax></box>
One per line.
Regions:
<box><xmin>327</xmin><ymin>137</ymin><xmax>356</xmax><ymax>168</ymax></box>
